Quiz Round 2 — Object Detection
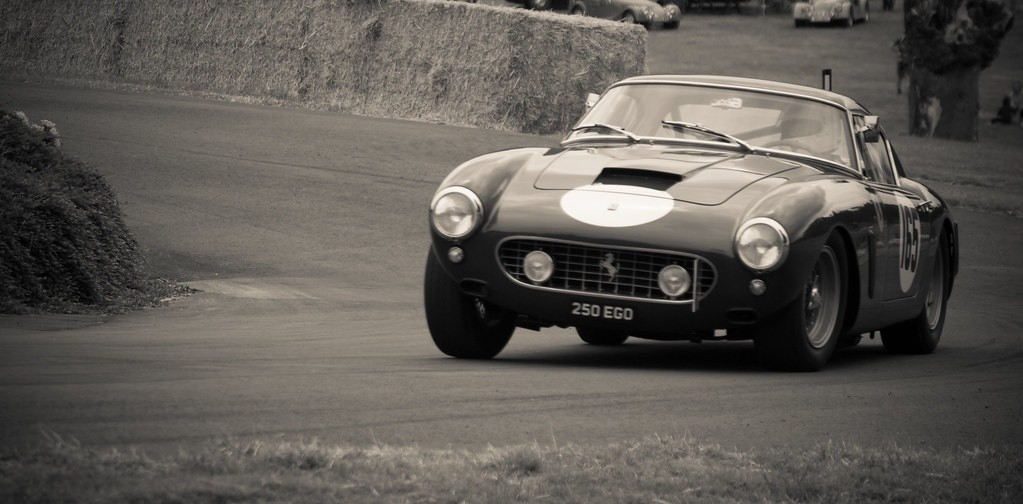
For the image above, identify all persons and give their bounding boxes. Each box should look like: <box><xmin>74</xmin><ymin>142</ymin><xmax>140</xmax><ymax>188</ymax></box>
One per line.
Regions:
<box><xmin>990</xmin><ymin>81</ymin><xmax>1023</xmax><ymax>127</ymax></box>
<box><xmin>776</xmin><ymin>105</ymin><xmax>842</xmax><ymax>163</ymax></box>
<box><xmin>890</xmin><ymin>30</ymin><xmax>909</xmax><ymax>94</ymax></box>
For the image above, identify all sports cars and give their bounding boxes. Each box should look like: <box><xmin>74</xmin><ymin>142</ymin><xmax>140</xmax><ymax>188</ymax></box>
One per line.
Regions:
<box><xmin>790</xmin><ymin>0</ymin><xmax>871</xmax><ymax>28</ymax></box>
<box><xmin>422</xmin><ymin>73</ymin><xmax>964</xmax><ymax>374</ymax></box>
<box><xmin>524</xmin><ymin>0</ymin><xmax>683</xmax><ymax>31</ymax></box>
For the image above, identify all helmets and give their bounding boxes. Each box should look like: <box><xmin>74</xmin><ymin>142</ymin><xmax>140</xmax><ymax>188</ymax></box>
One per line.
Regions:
<box><xmin>778</xmin><ymin>106</ymin><xmax>841</xmax><ymax>157</ymax></box>
